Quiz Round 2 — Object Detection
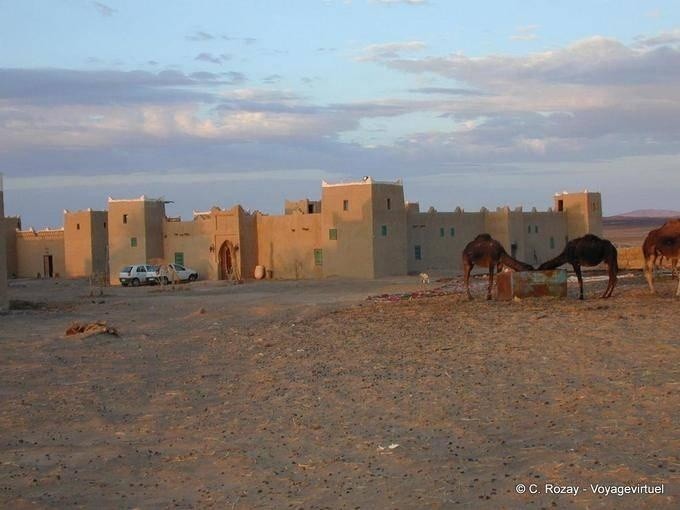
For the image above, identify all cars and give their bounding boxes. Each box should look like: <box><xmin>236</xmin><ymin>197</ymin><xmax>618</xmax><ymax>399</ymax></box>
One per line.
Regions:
<box><xmin>145</xmin><ymin>264</ymin><xmax>198</xmax><ymax>285</ymax></box>
<box><xmin>119</xmin><ymin>264</ymin><xmax>152</xmax><ymax>287</ymax></box>
